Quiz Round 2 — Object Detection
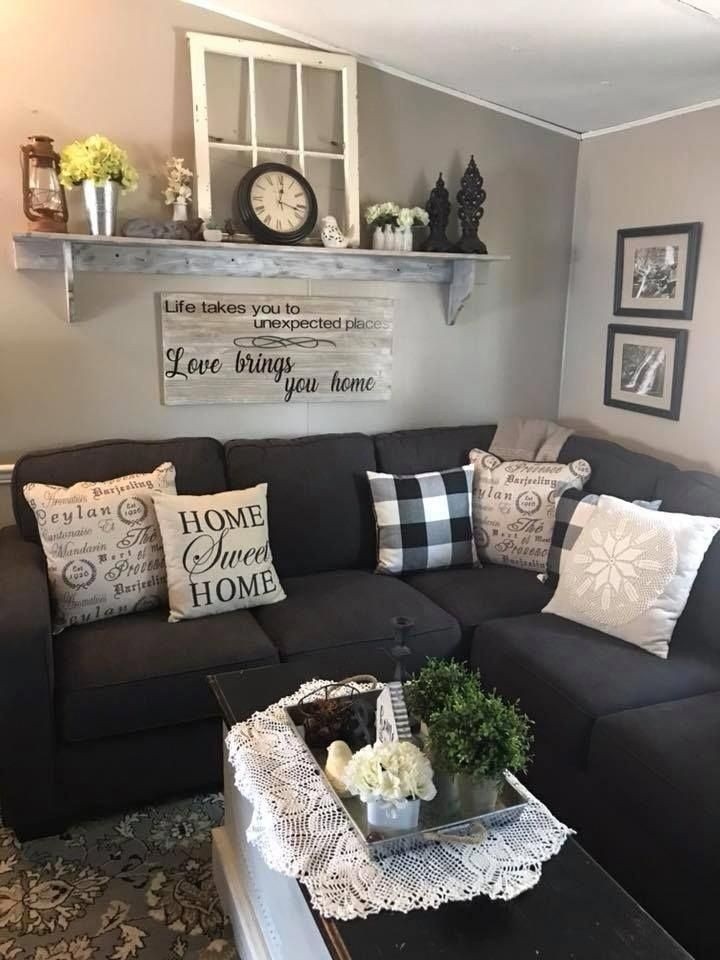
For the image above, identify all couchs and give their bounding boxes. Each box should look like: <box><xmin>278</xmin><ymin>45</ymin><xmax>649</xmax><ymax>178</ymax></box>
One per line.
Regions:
<box><xmin>0</xmin><ymin>425</ymin><xmax>720</xmax><ymax>960</ymax></box>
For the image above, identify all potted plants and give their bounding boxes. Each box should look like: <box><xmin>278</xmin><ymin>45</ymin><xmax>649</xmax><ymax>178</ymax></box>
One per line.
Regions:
<box><xmin>204</xmin><ymin>217</ymin><xmax>223</xmax><ymax>242</ymax></box>
<box><xmin>403</xmin><ymin>656</ymin><xmax>533</xmax><ymax>806</ymax></box>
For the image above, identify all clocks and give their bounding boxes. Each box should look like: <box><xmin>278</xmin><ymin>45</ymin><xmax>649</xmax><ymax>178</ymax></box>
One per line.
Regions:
<box><xmin>237</xmin><ymin>162</ymin><xmax>318</xmax><ymax>245</ymax></box>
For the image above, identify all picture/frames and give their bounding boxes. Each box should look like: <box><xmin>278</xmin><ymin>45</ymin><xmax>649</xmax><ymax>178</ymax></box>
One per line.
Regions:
<box><xmin>613</xmin><ymin>221</ymin><xmax>702</xmax><ymax>320</ymax></box>
<box><xmin>603</xmin><ymin>324</ymin><xmax>689</xmax><ymax>420</ymax></box>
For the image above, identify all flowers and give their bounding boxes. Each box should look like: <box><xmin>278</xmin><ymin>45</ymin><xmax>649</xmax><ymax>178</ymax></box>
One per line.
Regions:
<box><xmin>365</xmin><ymin>202</ymin><xmax>430</xmax><ymax>226</ymax></box>
<box><xmin>58</xmin><ymin>135</ymin><xmax>138</xmax><ymax>196</ymax></box>
<box><xmin>341</xmin><ymin>739</ymin><xmax>439</xmax><ymax>811</ymax></box>
<box><xmin>160</xmin><ymin>156</ymin><xmax>194</xmax><ymax>205</ymax></box>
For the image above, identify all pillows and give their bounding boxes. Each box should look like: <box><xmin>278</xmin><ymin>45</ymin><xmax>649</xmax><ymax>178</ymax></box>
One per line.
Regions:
<box><xmin>23</xmin><ymin>462</ymin><xmax>178</xmax><ymax>636</ymax></box>
<box><xmin>365</xmin><ymin>448</ymin><xmax>720</xmax><ymax>658</ymax></box>
<box><xmin>150</xmin><ymin>483</ymin><xmax>286</xmax><ymax>622</ymax></box>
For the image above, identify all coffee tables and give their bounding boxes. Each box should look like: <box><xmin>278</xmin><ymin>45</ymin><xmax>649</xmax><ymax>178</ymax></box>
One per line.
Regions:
<box><xmin>206</xmin><ymin>647</ymin><xmax>694</xmax><ymax>960</ymax></box>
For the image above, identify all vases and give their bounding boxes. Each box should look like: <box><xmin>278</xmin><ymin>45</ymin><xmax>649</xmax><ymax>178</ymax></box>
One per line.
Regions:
<box><xmin>367</xmin><ymin>797</ymin><xmax>421</xmax><ymax>829</ymax></box>
<box><xmin>173</xmin><ymin>202</ymin><xmax>187</xmax><ymax>221</ymax></box>
<box><xmin>81</xmin><ymin>180</ymin><xmax>119</xmax><ymax>236</ymax></box>
<box><xmin>373</xmin><ymin>224</ymin><xmax>413</xmax><ymax>251</ymax></box>
